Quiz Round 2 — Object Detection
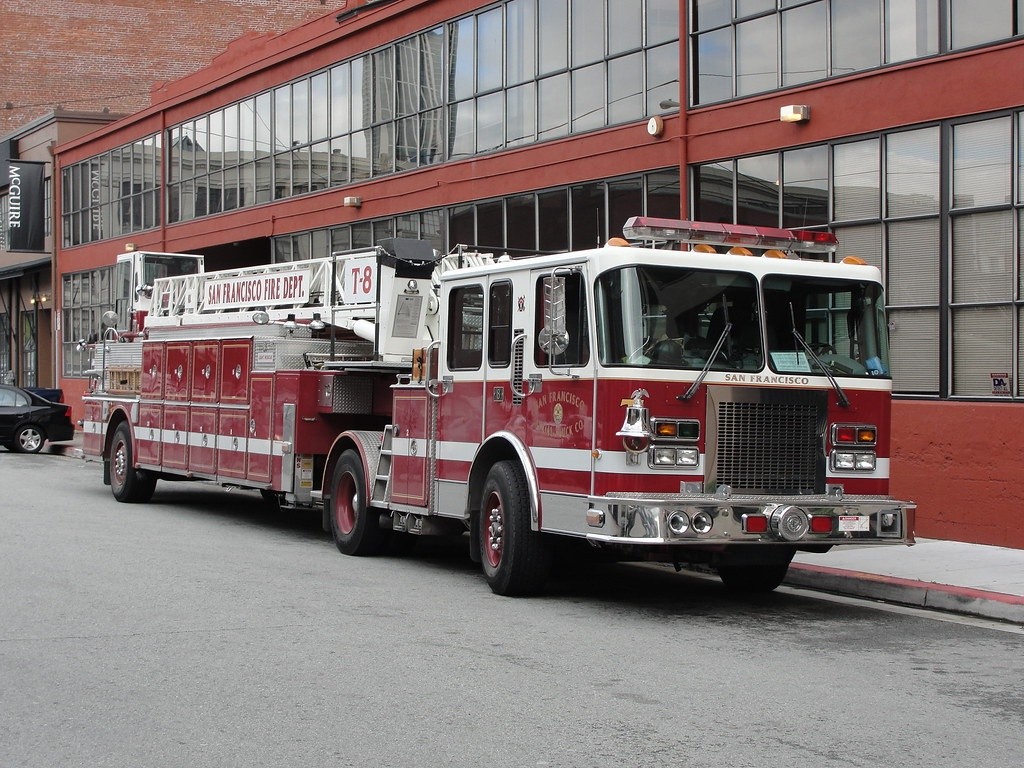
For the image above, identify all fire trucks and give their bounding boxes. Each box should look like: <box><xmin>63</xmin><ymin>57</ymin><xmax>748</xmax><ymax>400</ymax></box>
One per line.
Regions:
<box><xmin>77</xmin><ymin>215</ymin><xmax>917</xmax><ymax>598</ymax></box>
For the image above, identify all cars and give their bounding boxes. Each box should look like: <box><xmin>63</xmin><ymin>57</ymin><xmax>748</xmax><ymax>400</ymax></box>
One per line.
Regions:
<box><xmin>0</xmin><ymin>383</ymin><xmax>74</xmax><ymax>454</ymax></box>
